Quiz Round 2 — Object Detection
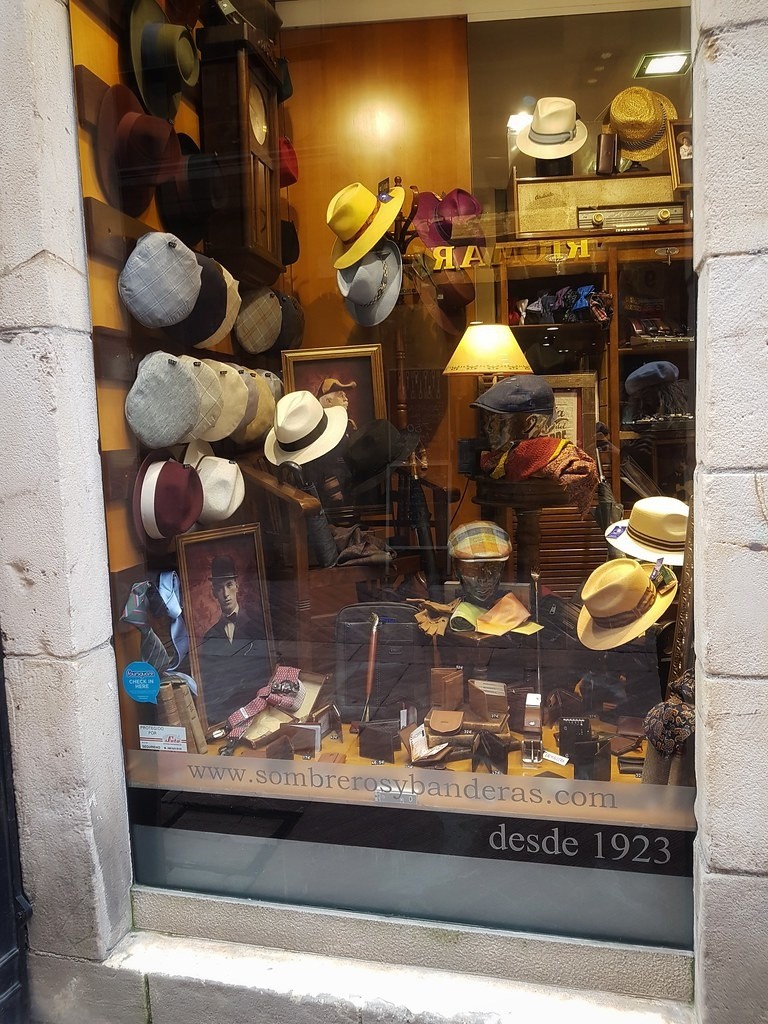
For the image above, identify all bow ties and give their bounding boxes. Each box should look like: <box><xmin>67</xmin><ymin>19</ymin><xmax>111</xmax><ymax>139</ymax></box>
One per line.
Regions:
<box><xmin>509</xmin><ymin>284</ymin><xmax>595</xmax><ymax>326</ymax></box>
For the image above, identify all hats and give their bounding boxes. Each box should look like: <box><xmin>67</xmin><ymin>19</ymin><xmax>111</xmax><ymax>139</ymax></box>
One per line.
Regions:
<box><xmin>594</xmin><ymin>85</ymin><xmax>680</xmax><ymax>163</ymax></box>
<box><xmin>604</xmin><ymin>496</ymin><xmax>689</xmax><ymax>579</ymax></box>
<box><xmin>515</xmin><ymin>98</ymin><xmax>588</xmax><ymax>162</ymax></box>
<box><xmin>624</xmin><ymin>360</ymin><xmax>681</xmax><ymax>398</ymax></box>
<box><xmin>409</xmin><ymin>188</ymin><xmax>486</xmax><ymax>276</ymax></box>
<box><xmin>445</xmin><ymin>516</ymin><xmax>513</xmax><ymax>564</ymax></box>
<box><xmin>675</xmin><ymin>131</ymin><xmax>689</xmax><ymax>145</ymax></box>
<box><xmin>92</xmin><ymin>80</ymin><xmax>177</xmax><ymax>219</ymax></box>
<box><xmin>341</xmin><ymin>418</ymin><xmax>421</xmax><ymax>506</ymax></box>
<box><xmin>394</xmin><ymin>237</ymin><xmax>477</xmax><ymax>336</ymax></box>
<box><xmin>130</xmin><ymin>0</ymin><xmax>200</xmax><ymax>122</ymax></box>
<box><xmin>155</xmin><ymin>133</ymin><xmax>229</xmax><ymax>248</ymax></box>
<box><xmin>118</xmin><ymin>231</ymin><xmax>350</xmax><ymax>561</ymax></box>
<box><xmin>324</xmin><ymin>180</ymin><xmax>406</xmax><ymax>272</ymax></box>
<box><xmin>577</xmin><ymin>558</ymin><xmax>680</xmax><ymax>654</ymax></box>
<box><xmin>469</xmin><ymin>374</ymin><xmax>555</xmax><ymax>418</ymax></box>
<box><xmin>336</xmin><ymin>235</ymin><xmax>417</xmax><ymax>328</ymax></box>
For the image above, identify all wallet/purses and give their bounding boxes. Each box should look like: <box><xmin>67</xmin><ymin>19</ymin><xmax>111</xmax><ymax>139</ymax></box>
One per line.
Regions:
<box><xmin>573</xmin><ymin>737</ymin><xmax>612</xmax><ymax>782</ymax></box>
<box><xmin>310</xmin><ymin>699</ymin><xmax>343</xmax><ymax>744</ymax></box>
<box><xmin>617</xmin><ymin>715</ymin><xmax>645</xmax><ymax>737</ymax></box>
<box><xmin>547</xmin><ymin>684</ymin><xmax>584</xmax><ymax>723</ymax></box>
<box><xmin>608</xmin><ymin>737</ymin><xmax>641</xmax><ymax>755</ymax></box>
<box><xmin>557</xmin><ymin>716</ymin><xmax>593</xmax><ymax>763</ymax></box>
<box><xmin>317</xmin><ymin>751</ymin><xmax>348</xmax><ymax>762</ymax></box>
<box><xmin>468</xmin><ymin>675</ymin><xmax>509</xmax><ymax>722</ymax></box>
<box><xmin>470</xmin><ymin>728</ymin><xmax>510</xmax><ymax>774</ymax></box>
<box><xmin>428</xmin><ymin>710</ymin><xmax>464</xmax><ymax>736</ymax></box>
<box><xmin>508</xmin><ymin>682</ymin><xmax>536</xmax><ymax>734</ymax></box>
<box><xmin>430</xmin><ymin>666</ymin><xmax>464</xmax><ymax>710</ymax></box>
<box><xmin>266</xmin><ymin>735</ymin><xmax>292</xmax><ymax>758</ymax></box>
<box><xmin>392</xmin><ymin>722</ymin><xmax>452</xmax><ymax>764</ymax></box>
<box><xmin>617</xmin><ymin>755</ymin><xmax>647</xmax><ymax>773</ymax></box>
<box><xmin>279</xmin><ymin>722</ymin><xmax>324</xmax><ymax>759</ymax></box>
<box><xmin>358</xmin><ymin>717</ymin><xmax>402</xmax><ymax>766</ymax></box>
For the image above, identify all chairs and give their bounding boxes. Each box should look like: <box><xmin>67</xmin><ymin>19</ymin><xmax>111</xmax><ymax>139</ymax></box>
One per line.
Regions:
<box><xmin>235</xmin><ymin>449</ymin><xmax>460</xmax><ymax>673</ymax></box>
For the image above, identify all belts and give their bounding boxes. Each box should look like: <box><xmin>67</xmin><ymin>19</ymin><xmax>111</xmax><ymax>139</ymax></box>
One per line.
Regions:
<box><xmin>623</xmin><ymin>316</ymin><xmax>686</xmax><ymax>339</ymax></box>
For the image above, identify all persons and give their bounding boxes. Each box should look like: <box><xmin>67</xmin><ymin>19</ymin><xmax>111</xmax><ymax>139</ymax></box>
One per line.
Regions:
<box><xmin>199</xmin><ymin>554</ymin><xmax>275</xmax><ymax>727</ymax></box>
<box><xmin>471</xmin><ymin>380</ymin><xmax>555</xmax><ymax>452</ymax></box>
<box><xmin>314</xmin><ymin>379</ymin><xmax>358</xmax><ymax>509</ymax></box>
<box><xmin>620</xmin><ymin>359</ymin><xmax>688</xmax><ymax>434</ymax></box>
<box><xmin>679</xmin><ymin>136</ymin><xmax>693</xmax><ymax>160</ymax></box>
<box><xmin>446</xmin><ymin>519</ymin><xmax>513</xmax><ymax>609</ymax></box>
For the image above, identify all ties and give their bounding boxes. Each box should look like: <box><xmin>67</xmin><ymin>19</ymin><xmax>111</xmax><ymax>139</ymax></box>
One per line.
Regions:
<box><xmin>119</xmin><ymin>581</ymin><xmax>171</xmax><ymax>676</ymax></box>
<box><xmin>155</xmin><ymin>569</ymin><xmax>199</xmax><ymax>698</ymax></box>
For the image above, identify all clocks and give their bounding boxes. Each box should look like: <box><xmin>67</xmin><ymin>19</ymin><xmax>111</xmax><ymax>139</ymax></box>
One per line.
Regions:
<box><xmin>196</xmin><ymin>23</ymin><xmax>294</xmax><ymax>289</ymax></box>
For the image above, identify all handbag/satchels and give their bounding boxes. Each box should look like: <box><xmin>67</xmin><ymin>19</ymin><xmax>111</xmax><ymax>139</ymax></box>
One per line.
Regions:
<box><xmin>539</xmin><ymin>596</ymin><xmax>581</xmax><ymax>646</ymax></box>
<box><xmin>282</xmin><ymin>220</ymin><xmax>299</xmax><ymax>266</ymax></box>
<box><xmin>335</xmin><ymin>603</ymin><xmax>431</xmax><ymax>725</ymax></box>
<box><xmin>276</xmin><ymin>57</ymin><xmax>293</xmax><ymax>105</ymax></box>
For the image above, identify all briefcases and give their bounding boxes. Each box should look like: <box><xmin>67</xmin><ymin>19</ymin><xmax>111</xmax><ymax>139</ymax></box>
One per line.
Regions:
<box><xmin>278</xmin><ymin>134</ymin><xmax>301</xmax><ymax>191</ymax></box>
<box><xmin>216</xmin><ymin>1</ymin><xmax>284</xmax><ymax>51</ymax></box>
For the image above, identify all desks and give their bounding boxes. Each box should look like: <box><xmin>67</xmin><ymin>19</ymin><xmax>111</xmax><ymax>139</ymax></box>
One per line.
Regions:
<box><xmin>469</xmin><ymin>475</ymin><xmax>586</xmax><ymax>596</ymax></box>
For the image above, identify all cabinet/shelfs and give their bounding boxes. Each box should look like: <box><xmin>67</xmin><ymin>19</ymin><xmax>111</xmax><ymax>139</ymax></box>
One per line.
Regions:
<box><xmin>494</xmin><ymin>233</ymin><xmax>697</xmax><ymax>603</ymax></box>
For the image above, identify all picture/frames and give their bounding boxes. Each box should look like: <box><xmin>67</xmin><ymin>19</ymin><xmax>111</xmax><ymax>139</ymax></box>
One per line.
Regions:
<box><xmin>513</xmin><ymin>371</ymin><xmax>597</xmax><ymax>464</ymax></box>
<box><xmin>176</xmin><ymin>522</ymin><xmax>278</xmax><ymax>739</ymax></box>
<box><xmin>280</xmin><ymin>343</ymin><xmax>392</xmax><ymax>516</ymax></box>
<box><xmin>665</xmin><ymin>118</ymin><xmax>693</xmax><ymax>190</ymax></box>
<box><xmin>444</xmin><ymin>581</ymin><xmax>532</xmax><ymax>610</ymax></box>
